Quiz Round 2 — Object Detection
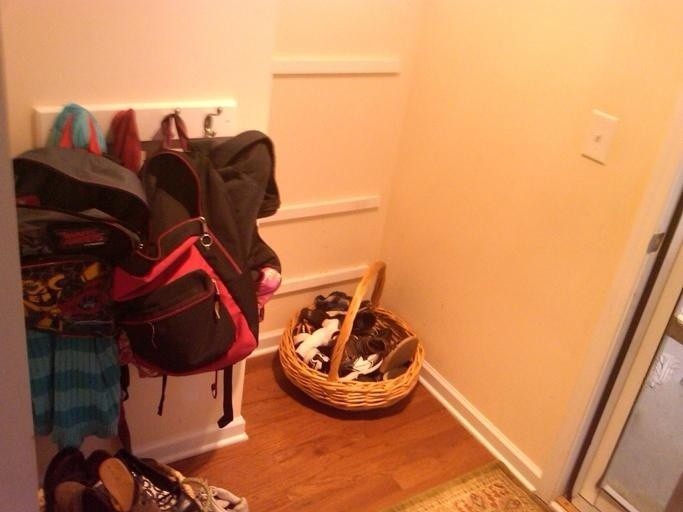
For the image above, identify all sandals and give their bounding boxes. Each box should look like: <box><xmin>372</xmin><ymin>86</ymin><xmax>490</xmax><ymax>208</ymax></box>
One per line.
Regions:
<box><xmin>292</xmin><ymin>290</ymin><xmax>419</xmax><ymax>382</ymax></box>
<box><xmin>42</xmin><ymin>444</ymin><xmax>202</xmax><ymax>511</ymax></box>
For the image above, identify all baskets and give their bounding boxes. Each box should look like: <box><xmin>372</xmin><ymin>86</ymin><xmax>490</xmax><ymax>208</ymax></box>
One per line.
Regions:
<box><xmin>278</xmin><ymin>260</ymin><xmax>426</xmax><ymax>413</ymax></box>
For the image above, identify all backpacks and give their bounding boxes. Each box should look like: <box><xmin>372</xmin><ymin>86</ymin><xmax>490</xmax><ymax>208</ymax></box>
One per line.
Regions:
<box><xmin>13</xmin><ymin>148</ymin><xmax>150</xmax><ymax>339</ymax></box>
<box><xmin>125</xmin><ymin>129</ymin><xmax>283</xmax><ymax>377</ymax></box>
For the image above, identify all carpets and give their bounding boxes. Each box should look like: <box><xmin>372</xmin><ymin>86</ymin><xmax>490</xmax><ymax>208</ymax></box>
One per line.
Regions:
<box><xmin>381</xmin><ymin>459</ymin><xmax>554</xmax><ymax>512</ymax></box>
